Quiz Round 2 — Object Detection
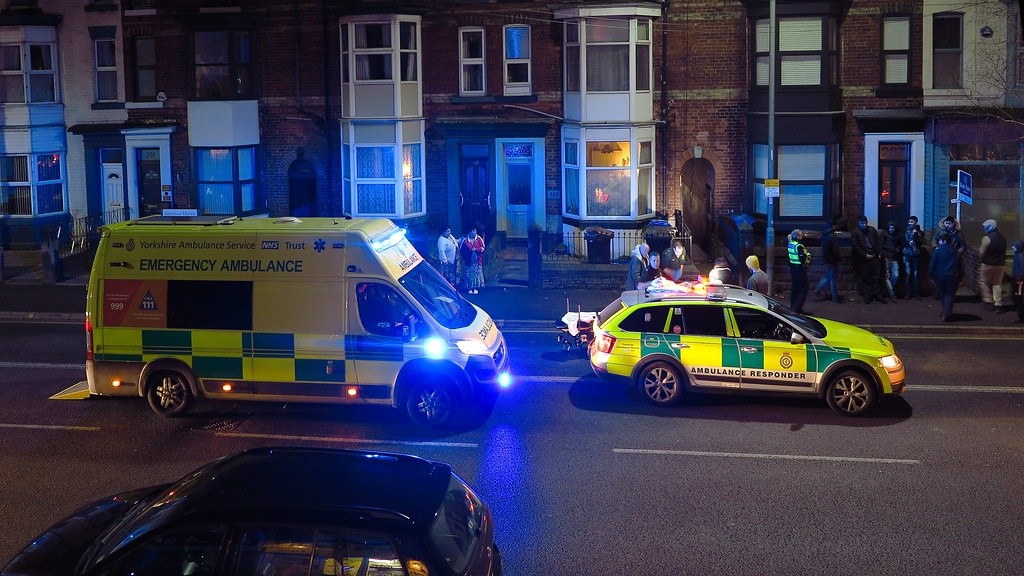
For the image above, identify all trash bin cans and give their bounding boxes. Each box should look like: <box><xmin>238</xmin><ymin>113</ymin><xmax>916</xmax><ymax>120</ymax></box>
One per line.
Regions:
<box><xmin>832</xmin><ymin>231</ymin><xmax>851</xmax><ymax>246</ymax></box>
<box><xmin>730</xmin><ymin>213</ymin><xmax>756</xmax><ymax>253</ymax></box>
<box><xmin>642</xmin><ymin>228</ymin><xmax>674</xmax><ymax>257</ymax></box>
<box><xmin>584</xmin><ymin>225</ymin><xmax>614</xmax><ymax>263</ymax></box>
<box><xmin>802</xmin><ymin>230</ymin><xmax>822</xmax><ymax>247</ymax></box>
<box><xmin>647</xmin><ymin>219</ymin><xmax>671</xmax><ymax>227</ymax></box>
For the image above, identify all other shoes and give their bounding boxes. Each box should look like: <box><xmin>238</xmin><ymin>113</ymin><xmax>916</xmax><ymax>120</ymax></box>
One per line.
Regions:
<box><xmin>904</xmin><ymin>295</ymin><xmax>911</xmax><ymax>299</ymax></box>
<box><xmin>469</xmin><ymin>290</ymin><xmax>473</xmax><ymax>294</ymax></box>
<box><xmin>880</xmin><ymin>298</ymin><xmax>887</xmax><ymax>303</ymax></box>
<box><xmin>813</xmin><ymin>290</ymin><xmax>824</xmax><ymax>300</ymax></box>
<box><xmin>833</xmin><ymin>300</ymin><xmax>846</xmax><ymax>304</ymax></box>
<box><xmin>916</xmin><ymin>295</ymin><xmax>922</xmax><ymax>300</ymax></box>
<box><xmin>997</xmin><ymin>307</ymin><xmax>1005</xmax><ymax>313</ymax></box>
<box><xmin>474</xmin><ymin>290</ymin><xmax>478</xmax><ymax>294</ymax></box>
<box><xmin>985</xmin><ymin>304</ymin><xmax>993</xmax><ymax>310</ymax></box>
<box><xmin>864</xmin><ymin>298</ymin><xmax>870</xmax><ymax>304</ymax></box>
<box><xmin>940</xmin><ymin>312</ymin><xmax>950</xmax><ymax>321</ymax></box>
<box><xmin>891</xmin><ymin>297</ymin><xmax>896</xmax><ymax>302</ymax></box>
<box><xmin>1011</xmin><ymin>317</ymin><xmax>1023</xmax><ymax>323</ymax></box>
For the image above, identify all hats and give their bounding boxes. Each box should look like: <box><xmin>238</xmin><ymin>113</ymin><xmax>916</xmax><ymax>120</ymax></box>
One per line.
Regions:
<box><xmin>746</xmin><ymin>255</ymin><xmax>759</xmax><ymax>268</ymax></box>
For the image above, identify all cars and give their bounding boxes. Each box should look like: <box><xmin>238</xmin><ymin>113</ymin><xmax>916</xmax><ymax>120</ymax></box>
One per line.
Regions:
<box><xmin>0</xmin><ymin>446</ymin><xmax>504</xmax><ymax>576</ymax></box>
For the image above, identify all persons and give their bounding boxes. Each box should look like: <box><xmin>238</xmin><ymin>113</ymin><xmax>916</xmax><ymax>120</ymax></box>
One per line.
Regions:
<box><xmin>850</xmin><ymin>214</ymin><xmax>1024</xmax><ymax>322</ymax></box>
<box><xmin>815</xmin><ymin>219</ymin><xmax>844</xmax><ymax>303</ymax></box>
<box><xmin>438</xmin><ymin>226</ymin><xmax>485</xmax><ymax>295</ymax></box>
<box><xmin>745</xmin><ymin>255</ymin><xmax>768</xmax><ymax>294</ymax></box>
<box><xmin>710</xmin><ymin>256</ymin><xmax>735</xmax><ymax>286</ymax></box>
<box><xmin>627</xmin><ymin>239</ymin><xmax>690</xmax><ymax>291</ymax></box>
<box><xmin>788</xmin><ymin>229</ymin><xmax>812</xmax><ymax>314</ymax></box>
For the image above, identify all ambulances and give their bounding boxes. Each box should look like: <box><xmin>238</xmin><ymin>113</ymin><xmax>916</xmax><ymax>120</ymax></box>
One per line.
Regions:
<box><xmin>587</xmin><ymin>280</ymin><xmax>907</xmax><ymax>417</ymax></box>
<box><xmin>85</xmin><ymin>215</ymin><xmax>511</xmax><ymax>433</ymax></box>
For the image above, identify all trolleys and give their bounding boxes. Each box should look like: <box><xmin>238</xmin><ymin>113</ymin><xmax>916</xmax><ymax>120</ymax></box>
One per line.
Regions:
<box><xmin>555</xmin><ymin>298</ymin><xmax>601</xmax><ymax>355</ymax></box>
